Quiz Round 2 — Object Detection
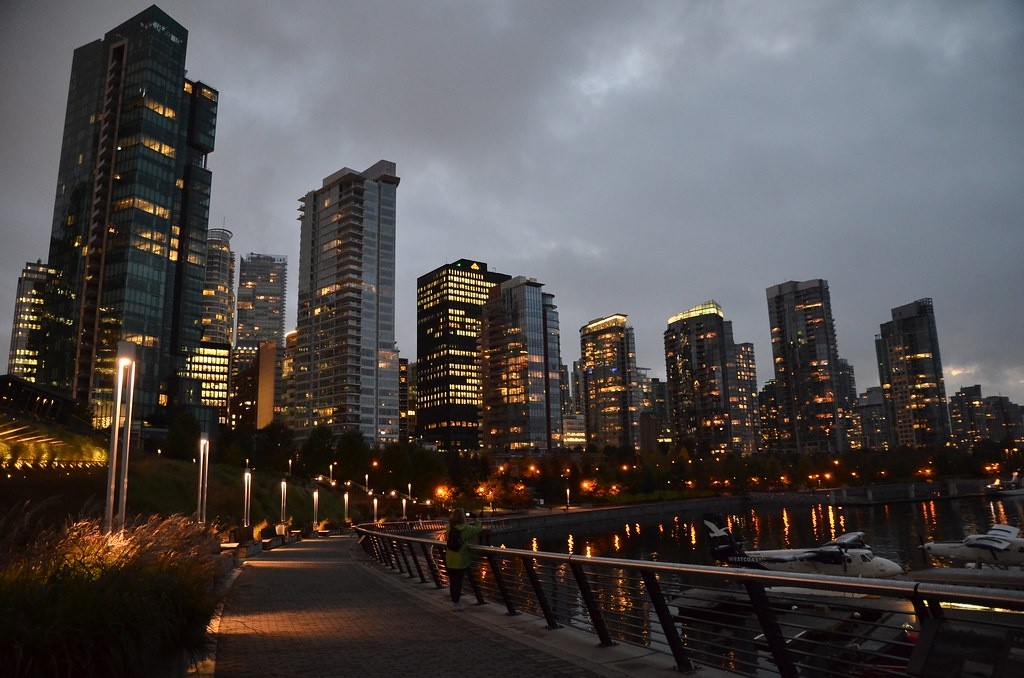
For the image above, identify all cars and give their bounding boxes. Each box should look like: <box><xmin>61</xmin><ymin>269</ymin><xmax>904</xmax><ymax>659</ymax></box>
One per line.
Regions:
<box><xmin>987</xmin><ymin>479</ymin><xmax>1019</xmax><ymax>490</ymax></box>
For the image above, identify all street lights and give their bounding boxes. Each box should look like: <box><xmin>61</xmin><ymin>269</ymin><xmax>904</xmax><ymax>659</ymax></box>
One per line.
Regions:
<box><xmin>196</xmin><ymin>431</ymin><xmax>210</xmax><ymax>523</ymax></box>
<box><xmin>343</xmin><ymin>491</ymin><xmax>349</xmax><ymax>517</ymax></box>
<box><xmin>312</xmin><ymin>489</ymin><xmax>319</xmax><ymax>522</ymax></box>
<box><xmin>281</xmin><ymin>477</ymin><xmax>287</xmax><ymax>522</ymax></box>
<box><xmin>329</xmin><ymin>463</ymin><xmax>334</xmax><ymax>478</ymax></box>
<box><xmin>288</xmin><ymin>458</ymin><xmax>293</xmax><ymax>476</ymax></box>
<box><xmin>408</xmin><ymin>483</ymin><xmax>411</xmax><ymax>500</ymax></box>
<box><xmin>243</xmin><ymin>467</ymin><xmax>252</xmax><ymax>526</ymax></box>
<box><xmin>401</xmin><ymin>498</ymin><xmax>408</xmax><ymax>519</ymax></box>
<box><xmin>102</xmin><ymin>340</ymin><xmax>137</xmax><ymax>536</ymax></box>
<box><xmin>372</xmin><ymin>497</ymin><xmax>379</xmax><ymax>523</ymax></box>
<box><xmin>364</xmin><ymin>474</ymin><xmax>369</xmax><ymax>492</ymax></box>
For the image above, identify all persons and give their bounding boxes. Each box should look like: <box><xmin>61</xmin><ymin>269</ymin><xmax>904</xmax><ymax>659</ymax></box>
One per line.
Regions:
<box><xmin>445</xmin><ymin>507</ymin><xmax>482</xmax><ymax>612</ymax></box>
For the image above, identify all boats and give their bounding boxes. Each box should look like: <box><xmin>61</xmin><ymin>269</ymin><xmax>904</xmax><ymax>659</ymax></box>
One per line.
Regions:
<box><xmin>666</xmin><ymin>523</ymin><xmax>1024</xmax><ymax>652</ymax></box>
